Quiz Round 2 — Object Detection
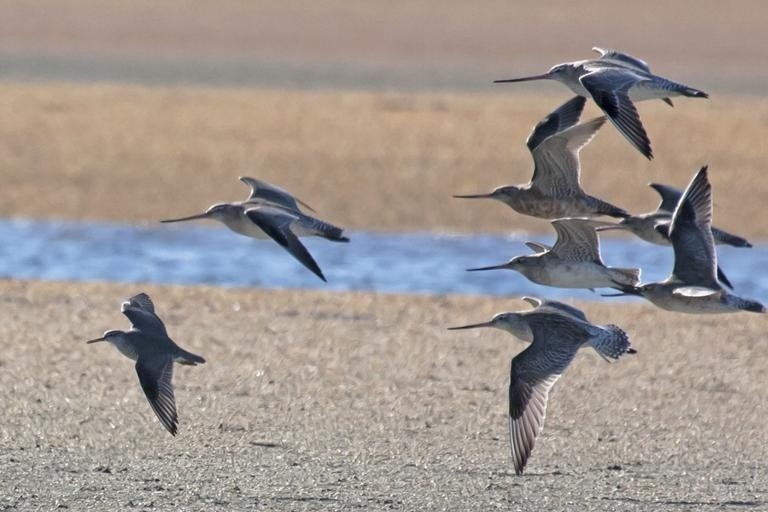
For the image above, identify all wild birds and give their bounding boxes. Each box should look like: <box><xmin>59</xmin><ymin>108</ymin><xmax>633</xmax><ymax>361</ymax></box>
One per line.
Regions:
<box><xmin>450</xmin><ymin>94</ymin><xmax>765</xmax><ymax>312</ymax></box>
<box><xmin>446</xmin><ymin>295</ymin><xmax>639</xmax><ymax>476</ymax></box>
<box><xmin>491</xmin><ymin>46</ymin><xmax>708</xmax><ymax>160</ymax></box>
<box><xmin>86</xmin><ymin>291</ymin><xmax>207</xmax><ymax>437</ymax></box>
<box><xmin>161</xmin><ymin>174</ymin><xmax>351</xmax><ymax>282</ymax></box>
